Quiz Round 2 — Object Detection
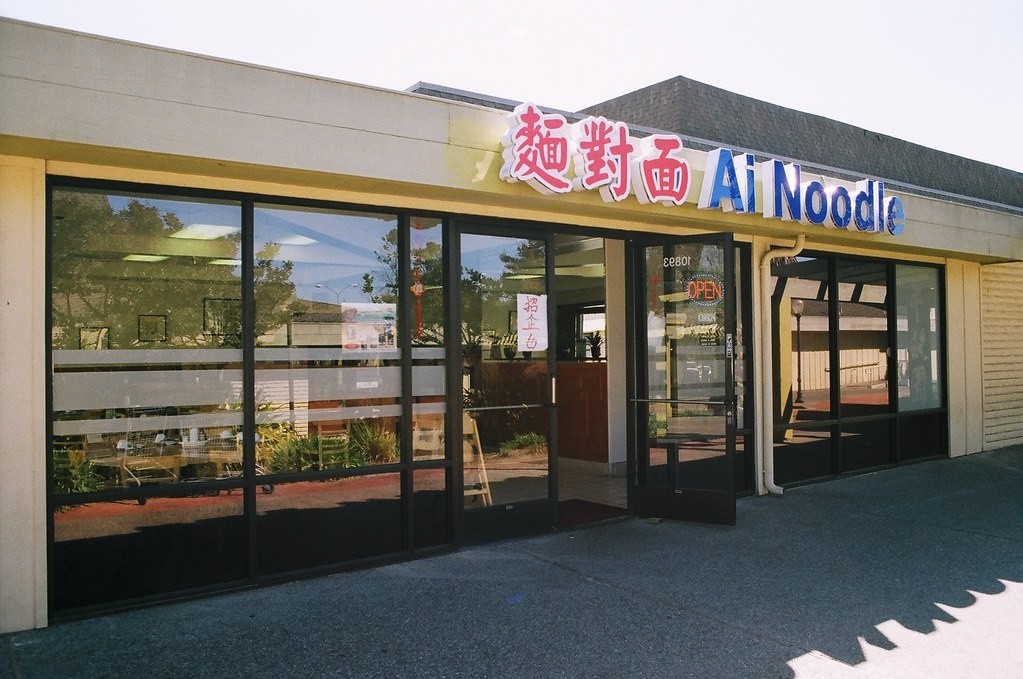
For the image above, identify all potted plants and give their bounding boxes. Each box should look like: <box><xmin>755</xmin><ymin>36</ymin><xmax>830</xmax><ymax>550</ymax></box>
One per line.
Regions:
<box><xmin>490</xmin><ymin>333</ymin><xmax>532</xmax><ymax>359</ymax></box>
<box><xmin>584</xmin><ymin>331</ymin><xmax>603</xmax><ymax>359</ymax></box>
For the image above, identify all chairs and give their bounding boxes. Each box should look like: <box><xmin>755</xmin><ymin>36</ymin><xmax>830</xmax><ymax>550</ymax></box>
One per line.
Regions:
<box><xmin>413</xmin><ymin>413</ymin><xmax>492</xmax><ymax>506</ymax></box>
<box><xmin>67</xmin><ymin>408</ymin><xmax>350</xmax><ymax>489</ymax></box>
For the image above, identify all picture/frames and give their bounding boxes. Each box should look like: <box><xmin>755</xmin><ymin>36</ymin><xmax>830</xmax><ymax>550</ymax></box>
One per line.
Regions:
<box><xmin>79</xmin><ymin>327</ymin><xmax>110</xmax><ymax>351</ymax></box>
<box><xmin>137</xmin><ymin>315</ymin><xmax>167</xmax><ymax>342</ymax></box>
<box><xmin>202</xmin><ymin>298</ymin><xmax>246</xmax><ymax>336</ymax></box>
<box><xmin>480</xmin><ymin>329</ymin><xmax>496</xmax><ymax>351</ymax></box>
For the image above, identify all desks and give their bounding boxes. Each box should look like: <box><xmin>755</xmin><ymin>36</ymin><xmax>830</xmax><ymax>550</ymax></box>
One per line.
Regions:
<box><xmin>88</xmin><ymin>447</ymin><xmax>208</xmax><ymax>466</ymax></box>
